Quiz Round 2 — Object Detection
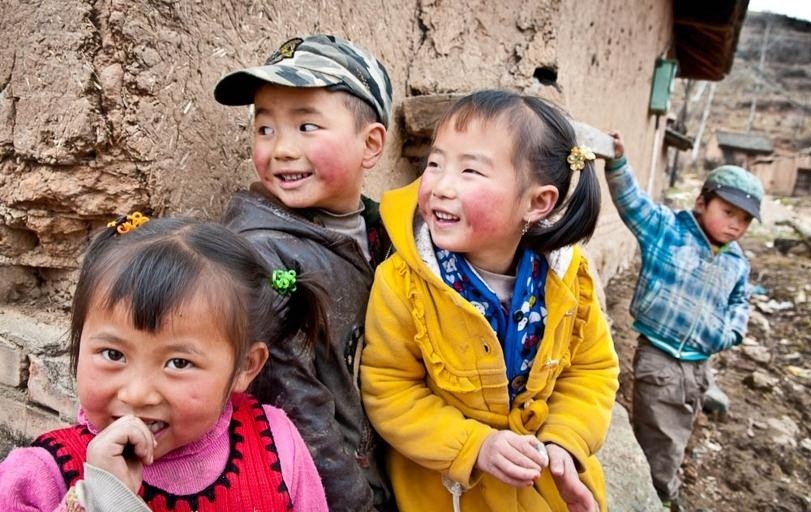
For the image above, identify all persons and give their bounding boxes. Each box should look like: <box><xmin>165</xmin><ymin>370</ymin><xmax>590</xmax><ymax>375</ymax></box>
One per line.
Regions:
<box><xmin>358</xmin><ymin>90</ymin><xmax>621</xmax><ymax>511</ymax></box>
<box><xmin>606</xmin><ymin>132</ymin><xmax>764</xmax><ymax>512</ymax></box>
<box><xmin>210</xmin><ymin>33</ymin><xmax>405</xmax><ymax>511</ymax></box>
<box><xmin>0</xmin><ymin>212</ymin><xmax>329</xmax><ymax>512</ymax></box>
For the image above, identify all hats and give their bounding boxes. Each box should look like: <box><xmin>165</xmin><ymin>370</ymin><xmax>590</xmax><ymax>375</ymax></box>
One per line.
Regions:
<box><xmin>214</xmin><ymin>33</ymin><xmax>392</xmax><ymax>131</ymax></box>
<box><xmin>701</xmin><ymin>164</ymin><xmax>765</xmax><ymax>223</ymax></box>
